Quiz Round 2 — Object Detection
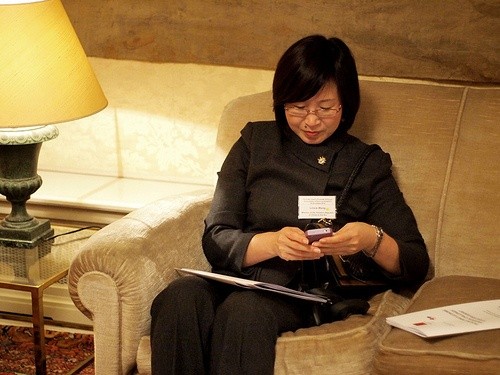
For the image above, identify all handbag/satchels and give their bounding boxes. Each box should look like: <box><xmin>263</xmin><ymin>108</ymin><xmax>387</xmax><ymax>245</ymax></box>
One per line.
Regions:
<box><xmin>304</xmin><ymin>144</ymin><xmax>398</xmax><ymax>300</ymax></box>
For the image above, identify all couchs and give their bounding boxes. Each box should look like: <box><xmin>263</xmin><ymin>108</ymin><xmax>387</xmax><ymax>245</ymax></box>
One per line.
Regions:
<box><xmin>68</xmin><ymin>79</ymin><xmax>500</xmax><ymax>375</ymax></box>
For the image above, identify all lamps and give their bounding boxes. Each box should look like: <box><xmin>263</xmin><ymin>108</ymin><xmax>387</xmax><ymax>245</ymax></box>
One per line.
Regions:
<box><xmin>1</xmin><ymin>1</ymin><xmax>109</xmax><ymax>250</ymax></box>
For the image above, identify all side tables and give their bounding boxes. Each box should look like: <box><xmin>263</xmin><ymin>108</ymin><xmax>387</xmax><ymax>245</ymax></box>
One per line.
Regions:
<box><xmin>0</xmin><ymin>224</ymin><xmax>97</xmax><ymax>375</ymax></box>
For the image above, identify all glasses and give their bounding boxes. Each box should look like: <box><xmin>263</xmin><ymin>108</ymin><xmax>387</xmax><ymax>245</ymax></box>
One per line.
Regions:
<box><xmin>284</xmin><ymin>104</ymin><xmax>342</xmax><ymax>118</ymax></box>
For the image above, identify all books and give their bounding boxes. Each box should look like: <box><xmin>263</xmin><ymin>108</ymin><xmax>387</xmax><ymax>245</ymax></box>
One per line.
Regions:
<box><xmin>385</xmin><ymin>300</ymin><xmax>500</xmax><ymax>338</ymax></box>
<box><xmin>174</xmin><ymin>268</ymin><xmax>328</xmax><ymax>303</ymax></box>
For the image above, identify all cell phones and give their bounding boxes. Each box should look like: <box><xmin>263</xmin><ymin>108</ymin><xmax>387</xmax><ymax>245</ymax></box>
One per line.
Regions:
<box><xmin>305</xmin><ymin>228</ymin><xmax>332</xmax><ymax>245</ymax></box>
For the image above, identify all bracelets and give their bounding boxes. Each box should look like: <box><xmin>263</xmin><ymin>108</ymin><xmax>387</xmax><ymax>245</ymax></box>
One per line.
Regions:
<box><xmin>362</xmin><ymin>224</ymin><xmax>384</xmax><ymax>258</ymax></box>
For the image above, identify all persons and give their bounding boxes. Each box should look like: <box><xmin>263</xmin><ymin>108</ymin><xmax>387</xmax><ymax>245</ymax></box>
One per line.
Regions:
<box><xmin>150</xmin><ymin>33</ymin><xmax>430</xmax><ymax>375</ymax></box>
